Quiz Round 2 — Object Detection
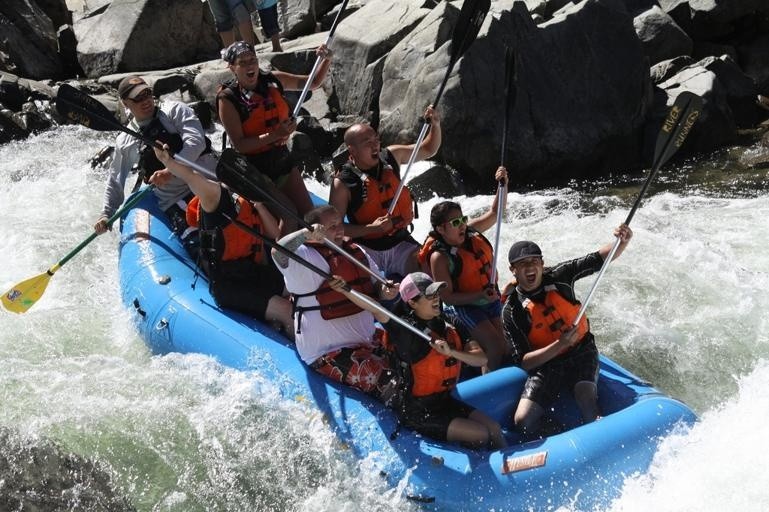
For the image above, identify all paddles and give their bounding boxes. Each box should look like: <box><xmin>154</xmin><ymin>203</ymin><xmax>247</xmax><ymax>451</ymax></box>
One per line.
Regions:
<box><xmin>573</xmin><ymin>91</ymin><xmax>704</xmax><ymax>326</ymax></box>
<box><xmin>55</xmin><ymin>84</ymin><xmax>218</xmax><ymax>185</ymax></box>
<box><xmin>222</xmin><ymin>211</ymin><xmax>434</xmax><ymax>344</ymax></box>
<box><xmin>387</xmin><ymin>0</ymin><xmax>491</xmax><ymax>214</ymax></box>
<box><xmin>1</xmin><ymin>182</ymin><xmax>155</xmax><ymax>315</ymax></box>
<box><xmin>485</xmin><ymin>46</ymin><xmax>515</xmax><ymax>292</ymax></box>
<box><xmin>214</xmin><ymin>148</ymin><xmax>395</xmax><ymax>288</ymax></box>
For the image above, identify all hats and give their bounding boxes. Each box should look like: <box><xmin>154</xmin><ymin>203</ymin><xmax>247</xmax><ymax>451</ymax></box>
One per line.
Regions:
<box><xmin>118</xmin><ymin>75</ymin><xmax>150</xmax><ymax>98</ymax></box>
<box><xmin>399</xmin><ymin>272</ymin><xmax>448</xmax><ymax>303</ymax></box>
<box><xmin>509</xmin><ymin>241</ymin><xmax>541</xmax><ymax>265</ymax></box>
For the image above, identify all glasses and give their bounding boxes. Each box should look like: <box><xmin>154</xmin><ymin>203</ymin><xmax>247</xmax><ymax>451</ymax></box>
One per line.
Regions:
<box><xmin>450</xmin><ymin>215</ymin><xmax>468</xmax><ymax>228</ymax></box>
<box><xmin>129</xmin><ymin>89</ymin><xmax>151</xmax><ymax>102</ymax></box>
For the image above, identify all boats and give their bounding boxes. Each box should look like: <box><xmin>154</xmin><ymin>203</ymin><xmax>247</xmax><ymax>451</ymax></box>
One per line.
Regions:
<box><xmin>119</xmin><ymin>180</ymin><xmax>703</xmax><ymax>511</ymax></box>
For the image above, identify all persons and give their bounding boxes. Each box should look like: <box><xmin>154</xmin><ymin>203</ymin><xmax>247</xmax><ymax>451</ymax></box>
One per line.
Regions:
<box><xmin>206</xmin><ymin>0</ymin><xmax>283</xmax><ymax>52</ymax></box>
<box><xmin>94</xmin><ymin>75</ymin><xmax>219</xmax><ymax>274</ymax></box>
<box><xmin>218</xmin><ymin>41</ymin><xmax>333</xmax><ymax>237</ymax></box>
<box><xmin>152</xmin><ymin>140</ymin><xmax>294</xmax><ymax>344</ymax></box>
<box><xmin>501</xmin><ymin>222</ymin><xmax>633</xmax><ymax>442</ymax></box>
<box><xmin>271</xmin><ymin>205</ymin><xmax>407</xmax><ymax>413</ymax></box>
<box><xmin>429</xmin><ymin>166</ymin><xmax>510</xmax><ymax>371</ymax></box>
<box><xmin>329</xmin><ymin>104</ymin><xmax>442</xmax><ymax>278</ymax></box>
<box><xmin>329</xmin><ymin>271</ymin><xmax>508</xmax><ymax>451</ymax></box>
